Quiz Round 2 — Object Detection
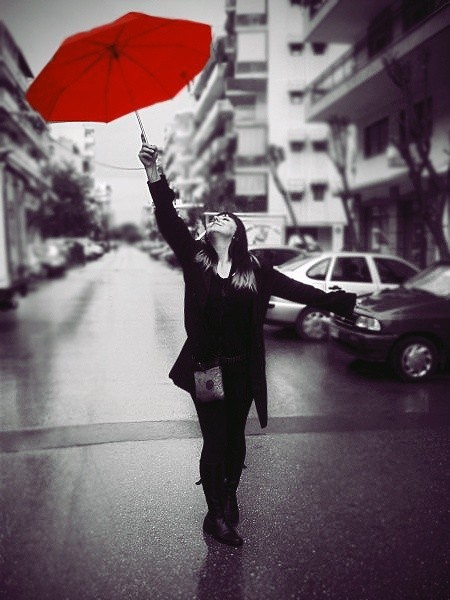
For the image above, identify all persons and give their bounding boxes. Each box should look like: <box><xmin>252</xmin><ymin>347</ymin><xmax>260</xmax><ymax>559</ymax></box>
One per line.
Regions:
<box><xmin>138</xmin><ymin>133</ymin><xmax>358</xmax><ymax>547</ymax></box>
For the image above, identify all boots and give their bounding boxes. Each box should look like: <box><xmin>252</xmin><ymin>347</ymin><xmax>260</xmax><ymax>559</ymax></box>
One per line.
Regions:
<box><xmin>222</xmin><ymin>456</ymin><xmax>247</xmax><ymax>530</ymax></box>
<box><xmin>196</xmin><ymin>465</ymin><xmax>242</xmax><ymax>546</ymax></box>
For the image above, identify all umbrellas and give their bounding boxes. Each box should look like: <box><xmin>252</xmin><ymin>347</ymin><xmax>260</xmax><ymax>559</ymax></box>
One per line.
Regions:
<box><xmin>25</xmin><ymin>11</ymin><xmax>212</xmax><ymax>161</ymax></box>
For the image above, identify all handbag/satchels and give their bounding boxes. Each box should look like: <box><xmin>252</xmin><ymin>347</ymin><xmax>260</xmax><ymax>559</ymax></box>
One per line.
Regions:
<box><xmin>193</xmin><ymin>365</ymin><xmax>225</xmax><ymax>404</ymax></box>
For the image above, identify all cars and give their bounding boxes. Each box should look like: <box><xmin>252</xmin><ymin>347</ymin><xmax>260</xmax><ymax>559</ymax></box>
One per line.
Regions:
<box><xmin>265</xmin><ymin>252</ymin><xmax>426</xmax><ymax>343</ymax></box>
<box><xmin>136</xmin><ymin>238</ymin><xmax>184</xmax><ymax>268</ymax></box>
<box><xmin>27</xmin><ymin>235</ymin><xmax>119</xmax><ymax>276</ymax></box>
<box><xmin>321</xmin><ymin>261</ymin><xmax>450</xmax><ymax>380</ymax></box>
<box><xmin>250</xmin><ymin>247</ymin><xmax>308</xmax><ymax>273</ymax></box>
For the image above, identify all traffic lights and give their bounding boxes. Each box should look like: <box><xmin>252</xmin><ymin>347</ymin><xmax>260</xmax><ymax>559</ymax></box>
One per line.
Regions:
<box><xmin>83</xmin><ymin>127</ymin><xmax>95</xmax><ymax>171</ymax></box>
